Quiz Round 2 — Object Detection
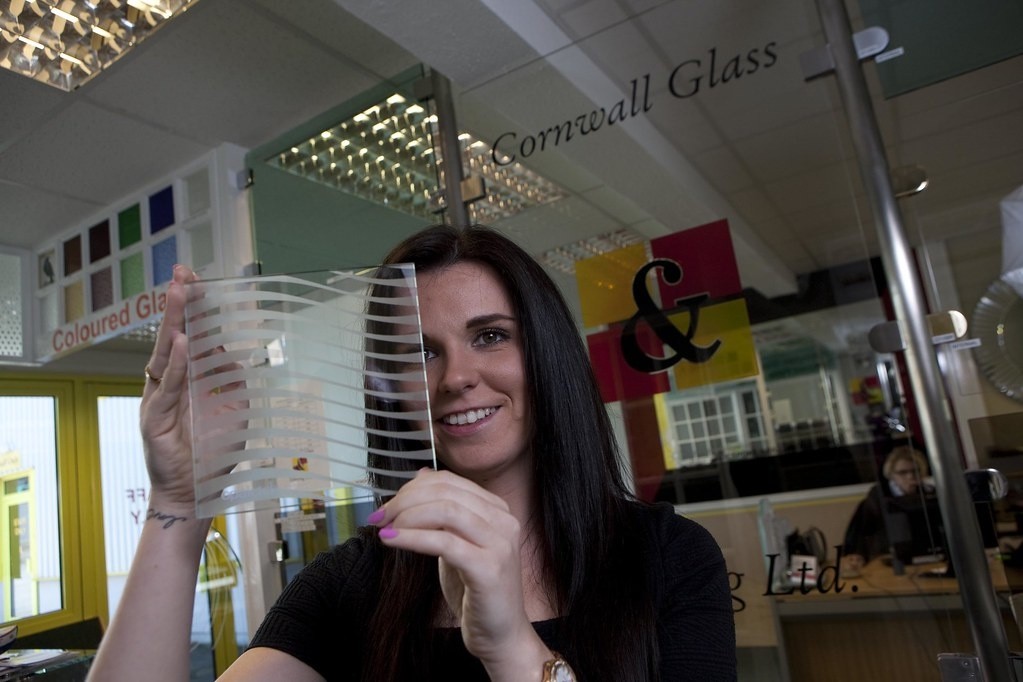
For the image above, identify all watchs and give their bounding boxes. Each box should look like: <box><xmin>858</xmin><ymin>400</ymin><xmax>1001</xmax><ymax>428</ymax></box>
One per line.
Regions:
<box><xmin>541</xmin><ymin>651</ymin><xmax>576</xmax><ymax>682</ymax></box>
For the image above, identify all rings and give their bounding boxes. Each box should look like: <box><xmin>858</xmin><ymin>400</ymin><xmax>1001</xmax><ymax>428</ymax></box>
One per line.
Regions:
<box><xmin>144</xmin><ymin>367</ymin><xmax>161</xmax><ymax>383</ymax></box>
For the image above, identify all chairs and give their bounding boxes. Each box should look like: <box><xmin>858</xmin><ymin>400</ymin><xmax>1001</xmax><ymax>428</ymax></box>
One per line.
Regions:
<box><xmin>984</xmin><ymin>446</ymin><xmax>1023</xmax><ymax>458</ymax></box>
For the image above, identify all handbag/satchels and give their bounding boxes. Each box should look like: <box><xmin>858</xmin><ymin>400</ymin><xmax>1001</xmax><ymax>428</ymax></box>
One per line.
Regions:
<box><xmin>785</xmin><ymin>526</ymin><xmax>826</xmax><ymax>565</ymax></box>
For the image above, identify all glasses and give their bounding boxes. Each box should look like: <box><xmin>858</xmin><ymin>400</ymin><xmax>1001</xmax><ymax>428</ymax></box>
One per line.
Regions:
<box><xmin>892</xmin><ymin>468</ymin><xmax>922</xmax><ymax>474</ymax></box>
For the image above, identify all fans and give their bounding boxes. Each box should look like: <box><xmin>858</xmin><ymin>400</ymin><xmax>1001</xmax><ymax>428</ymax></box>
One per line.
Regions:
<box><xmin>971</xmin><ymin>266</ymin><xmax>1023</xmax><ymax>404</ymax></box>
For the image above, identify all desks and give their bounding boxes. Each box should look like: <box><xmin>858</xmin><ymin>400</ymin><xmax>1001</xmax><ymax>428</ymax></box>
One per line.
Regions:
<box><xmin>981</xmin><ymin>455</ymin><xmax>1022</xmax><ymax>510</ymax></box>
<box><xmin>770</xmin><ymin>548</ymin><xmax>1023</xmax><ymax>682</ymax></box>
<box><xmin>0</xmin><ymin>649</ymin><xmax>97</xmax><ymax>682</ymax></box>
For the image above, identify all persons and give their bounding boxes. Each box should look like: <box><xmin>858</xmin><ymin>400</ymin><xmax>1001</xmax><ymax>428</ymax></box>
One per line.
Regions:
<box><xmin>87</xmin><ymin>223</ymin><xmax>736</xmax><ymax>682</ymax></box>
<box><xmin>843</xmin><ymin>449</ymin><xmax>937</xmax><ymax>569</ymax></box>
